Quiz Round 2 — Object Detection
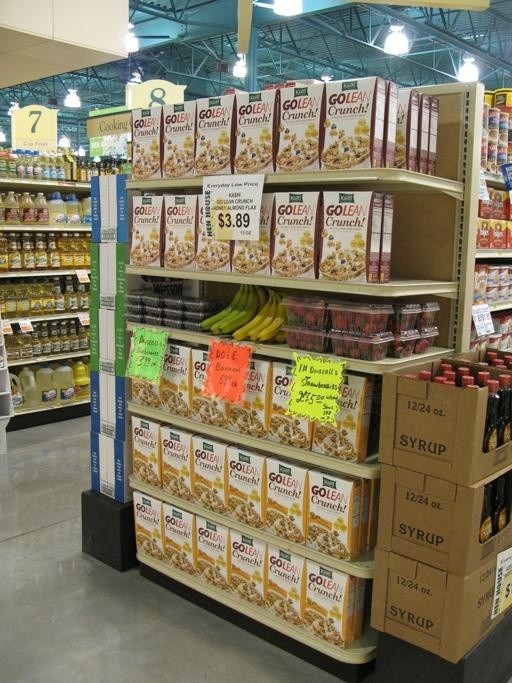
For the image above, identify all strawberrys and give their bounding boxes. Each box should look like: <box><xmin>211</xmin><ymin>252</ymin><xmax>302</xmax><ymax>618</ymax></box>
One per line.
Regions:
<box><xmin>282</xmin><ymin>294</ymin><xmax>441</xmax><ymax>361</ymax></box>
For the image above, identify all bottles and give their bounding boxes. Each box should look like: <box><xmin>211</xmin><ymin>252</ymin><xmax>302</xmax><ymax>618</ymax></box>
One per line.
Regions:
<box><xmin>0</xmin><ymin>143</ymin><xmax>129</xmax><ymax>183</ymax></box>
<box><xmin>1</xmin><ymin>275</ymin><xmax>89</xmax><ymax>313</ymax></box>
<box><xmin>0</xmin><ymin>189</ymin><xmax>93</xmax><ymax>225</ymax></box>
<box><xmin>0</xmin><ymin>232</ymin><xmax>91</xmax><ymax>269</ymax></box>
<box><xmin>472</xmin><ymin>471</ymin><xmax>508</xmax><ymax>541</ymax></box>
<box><xmin>400</xmin><ymin>347</ymin><xmax>512</xmax><ymax>450</ymax></box>
<box><xmin>2</xmin><ymin>319</ymin><xmax>91</xmax><ymax>359</ymax></box>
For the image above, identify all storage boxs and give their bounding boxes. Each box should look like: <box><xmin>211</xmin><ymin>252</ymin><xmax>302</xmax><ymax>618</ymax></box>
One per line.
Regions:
<box><xmin>374</xmin><ymin>462</ymin><xmax>510</xmax><ymax>576</ymax></box>
<box><xmin>369</xmin><ymin>350</ymin><xmax>511</xmax><ymax>488</ymax></box>
<box><xmin>370</xmin><ymin>546</ymin><xmax>511</xmax><ymax>665</ymax></box>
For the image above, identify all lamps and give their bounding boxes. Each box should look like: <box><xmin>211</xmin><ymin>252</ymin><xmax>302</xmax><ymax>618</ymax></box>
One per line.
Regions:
<box><xmin>383</xmin><ymin>25</ymin><xmax>408</xmax><ymax>55</ymax></box>
<box><xmin>128</xmin><ymin>22</ymin><xmax>139</xmax><ymax>53</ymax></box>
<box><xmin>233</xmin><ymin>54</ymin><xmax>246</xmax><ymax>78</ymax></box>
<box><xmin>457</xmin><ymin>54</ymin><xmax>478</xmax><ymax>83</ymax></box>
<box><xmin>64</xmin><ymin>73</ymin><xmax>80</xmax><ymax>108</ymax></box>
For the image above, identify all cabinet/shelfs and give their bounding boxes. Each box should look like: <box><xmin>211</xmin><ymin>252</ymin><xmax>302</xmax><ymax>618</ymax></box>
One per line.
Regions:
<box><xmin>124</xmin><ymin>80</ymin><xmax>512</xmax><ymax>683</ymax></box>
<box><xmin>0</xmin><ymin>178</ymin><xmax>90</xmax><ymax>417</ymax></box>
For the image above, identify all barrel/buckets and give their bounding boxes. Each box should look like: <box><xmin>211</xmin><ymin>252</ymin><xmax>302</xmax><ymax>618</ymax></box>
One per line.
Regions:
<box><xmin>9</xmin><ymin>356</ymin><xmax>91</xmax><ymax>409</ymax></box>
<box><xmin>58</xmin><ymin>233</ymin><xmax>91</xmax><ymax>268</ymax></box>
<box><xmin>0</xmin><ymin>192</ymin><xmax>92</xmax><ymax>226</ymax></box>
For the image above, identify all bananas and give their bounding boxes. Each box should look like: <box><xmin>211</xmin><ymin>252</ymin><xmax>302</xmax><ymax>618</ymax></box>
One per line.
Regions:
<box><xmin>198</xmin><ymin>284</ymin><xmax>267</xmax><ymax>335</ymax></box>
<box><xmin>232</xmin><ymin>287</ymin><xmax>289</xmax><ymax>345</ymax></box>
